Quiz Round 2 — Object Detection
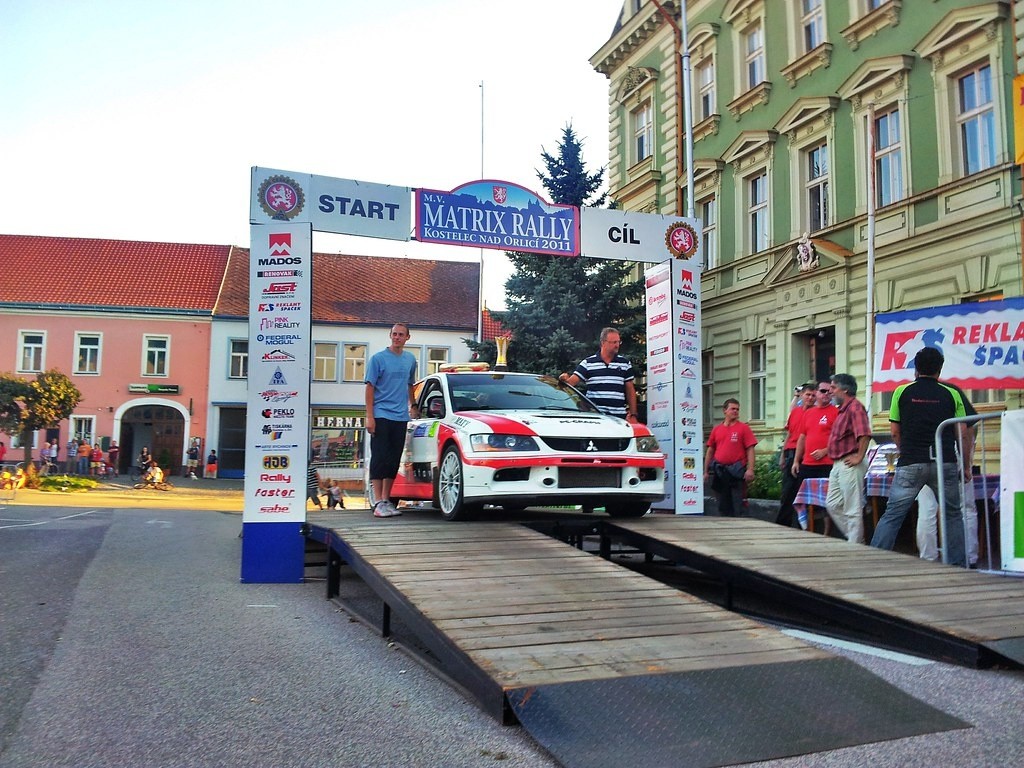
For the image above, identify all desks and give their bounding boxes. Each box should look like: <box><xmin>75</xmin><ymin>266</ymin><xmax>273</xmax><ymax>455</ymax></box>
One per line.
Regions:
<box><xmin>792</xmin><ymin>473</ymin><xmax>1000</xmax><ymax>557</ymax></box>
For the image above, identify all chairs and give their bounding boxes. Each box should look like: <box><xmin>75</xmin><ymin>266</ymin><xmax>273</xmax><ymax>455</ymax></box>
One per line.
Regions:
<box><xmin>426</xmin><ymin>396</ymin><xmax>478</xmax><ymax>417</ymax></box>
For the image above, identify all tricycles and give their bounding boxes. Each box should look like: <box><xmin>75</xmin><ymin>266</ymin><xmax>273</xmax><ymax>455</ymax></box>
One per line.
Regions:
<box><xmin>131</xmin><ymin>461</ymin><xmax>171</xmax><ymax>482</ymax></box>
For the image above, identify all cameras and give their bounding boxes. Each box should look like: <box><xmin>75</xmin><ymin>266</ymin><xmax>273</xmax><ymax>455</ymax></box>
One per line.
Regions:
<box><xmin>794</xmin><ymin>387</ymin><xmax>803</xmax><ymax>391</ymax></box>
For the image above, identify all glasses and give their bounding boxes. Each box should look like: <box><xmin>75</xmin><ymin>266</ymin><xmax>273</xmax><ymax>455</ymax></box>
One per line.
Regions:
<box><xmin>805</xmin><ymin>393</ymin><xmax>816</xmax><ymax>397</ymax></box>
<box><xmin>604</xmin><ymin>339</ymin><xmax>623</xmax><ymax>344</ymax></box>
<box><xmin>819</xmin><ymin>388</ymin><xmax>832</xmax><ymax>394</ymax></box>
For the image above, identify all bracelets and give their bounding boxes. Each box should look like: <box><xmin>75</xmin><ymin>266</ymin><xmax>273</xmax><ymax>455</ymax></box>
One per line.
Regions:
<box><xmin>630</xmin><ymin>414</ymin><xmax>636</xmax><ymax>417</ymax></box>
<box><xmin>794</xmin><ymin>395</ymin><xmax>800</xmax><ymax>397</ymax></box>
<box><xmin>411</xmin><ymin>404</ymin><xmax>418</xmax><ymax>409</ymax></box>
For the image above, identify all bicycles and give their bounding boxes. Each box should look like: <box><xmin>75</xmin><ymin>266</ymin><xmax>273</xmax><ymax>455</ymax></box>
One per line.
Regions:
<box><xmin>16</xmin><ymin>456</ymin><xmax>59</xmax><ymax>477</ymax></box>
<box><xmin>133</xmin><ymin>479</ymin><xmax>174</xmax><ymax>491</ymax></box>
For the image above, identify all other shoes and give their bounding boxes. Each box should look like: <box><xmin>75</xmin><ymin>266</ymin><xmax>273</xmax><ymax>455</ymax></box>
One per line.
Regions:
<box><xmin>583</xmin><ymin>506</ymin><xmax>594</xmax><ymax>513</ymax></box>
<box><xmin>969</xmin><ymin>563</ymin><xmax>978</xmax><ymax>569</ymax></box>
<box><xmin>184</xmin><ymin>474</ymin><xmax>189</xmax><ymax>478</ymax></box>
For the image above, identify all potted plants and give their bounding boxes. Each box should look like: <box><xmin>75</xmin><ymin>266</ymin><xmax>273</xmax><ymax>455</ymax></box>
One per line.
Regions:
<box><xmin>156</xmin><ymin>449</ymin><xmax>175</xmax><ymax>475</ymax></box>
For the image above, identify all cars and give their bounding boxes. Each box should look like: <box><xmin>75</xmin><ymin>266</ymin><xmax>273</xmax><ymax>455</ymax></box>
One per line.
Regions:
<box><xmin>364</xmin><ymin>362</ymin><xmax>665</xmax><ymax>520</ymax></box>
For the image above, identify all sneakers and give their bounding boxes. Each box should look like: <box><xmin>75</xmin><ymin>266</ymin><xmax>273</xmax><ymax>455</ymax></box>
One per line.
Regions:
<box><xmin>370</xmin><ymin>501</ymin><xmax>391</xmax><ymax>517</ymax></box>
<box><xmin>384</xmin><ymin>500</ymin><xmax>402</xmax><ymax>515</ymax></box>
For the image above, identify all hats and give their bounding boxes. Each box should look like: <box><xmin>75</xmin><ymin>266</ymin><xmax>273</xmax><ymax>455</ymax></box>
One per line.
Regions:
<box><xmin>72</xmin><ymin>437</ymin><xmax>78</xmax><ymax>441</ymax></box>
<box><xmin>801</xmin><ymin>379</ymin><xmax>818</xmax><ymax>387</ymax></box>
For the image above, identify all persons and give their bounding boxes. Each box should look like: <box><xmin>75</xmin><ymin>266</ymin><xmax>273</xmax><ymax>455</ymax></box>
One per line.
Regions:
<box><xmin>138</xmin><ymin>446</ymin><xmax>163</xmax><ymax>485</ymax></box>
<box><xmin>626</xmin><ymin>405</ymin><xmax>631</xmax><ymax>421</ymax></box>
<box><xmin>825</xmin><ymin>373</ymin><xmax>871</xmax><ymax>545</ymax></box>
<box><xmin>0</xmin><ymin>461</ymin><xmax>42</xmax><ymax>489</ymax></box>
<box><xmin>704</xmin><ymin>398</ymin><xmax>759</xmax><ymax>517</ymax></box>
<box><xmin>0</xmin><ymin>441</ymin><xmax>6</xmax><ymax>470</ymax></box>
<box><xmin>776</xmin><ymin>380</ymin><xmax>839</xmax><ymax>528</ymax></box>
<box><xmin>916</xmin><ymin>381</ymin><xmax>979</xmax><ymax>568</ymax></box>
<box><xmin>307</xmin><ymin>460</ymin><xmax>324</xmax><ymax>511</ymax></box>
<box><xmin>39</xmin><ymin>438</ymin><xmax>60</xmax><ymax>473</ymax></box>
<box><xmin>70</xmin><ymin>440</ymin><xmax>119</xmax><ymax>477</ymax></box>
<box><xmin>323</xmin><ymin>478</ymin><xmax>345</xmax><ymax>510</ymax></box>
<box><xmin>559</xmin><ymin>327</ymin><xmax>638</xmax><ymax>424</ymax></box>
<box><xmin>206</xmin><ymin>450</ymin><xmax>218</xmax><ymax>477</ymax></box>
<box><xmin>364</xmin><ymin>323</ymin><xmax>422</xmax><ymax>517</ymax></box>
<box><xmin>186</xmin><ymin>441</ymin><xmax>200</xmax><ymax>474</ymax></box>
<box><xmin>870</xmin><ymin>347</ymin><xmax>972</xmax><ymax>568</ymax></box>
